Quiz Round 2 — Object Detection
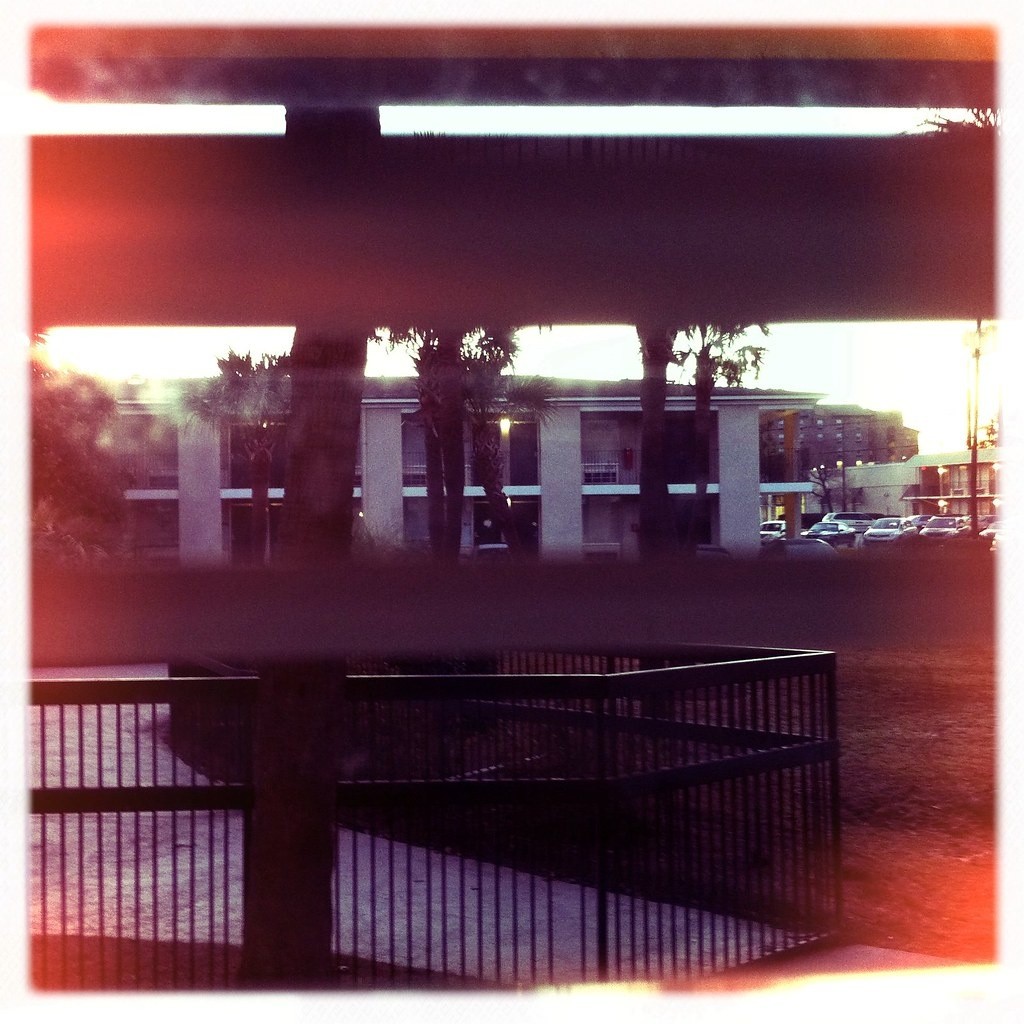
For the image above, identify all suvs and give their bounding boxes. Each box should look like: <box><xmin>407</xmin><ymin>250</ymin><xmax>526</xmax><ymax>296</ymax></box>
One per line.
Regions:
<box><xmin>821</xmin><ymin>510</ymin><xmax>876</xmax><ymax>534</ymax></box>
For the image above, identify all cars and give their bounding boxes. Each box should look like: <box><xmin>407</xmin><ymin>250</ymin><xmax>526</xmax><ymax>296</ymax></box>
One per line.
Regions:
<box><xmin>759</xmin><ymin>520</ymin><xmax>787</xmax><ymax>542</ymax></box>
<box><xmin>905</xmin><ymin>514</ymin><xmax>934</xmax><ymax>534</ymax></box>
<box><xmin>919</xmin><ymin>516</ymin><xmax>968</xmax><ymax>537</ymax></box>
<box><xmin>760</xmin><ymin>536</ymin><xmax>837</xmax><ymax>556</ymax></box>
<box><xmin>948</xmin><ymin>511</ymin><xmax>1003</xmax><ymax>537</ymax></box>
<box><xmin>863</xmin><ymin>516</ymin><xmax>917</xmax><ymax>543</ymax></box>
<box><xmin>800</xmin><ymin>521</ymin><xmax>858</xmax><ymax>548</ymax></box>
